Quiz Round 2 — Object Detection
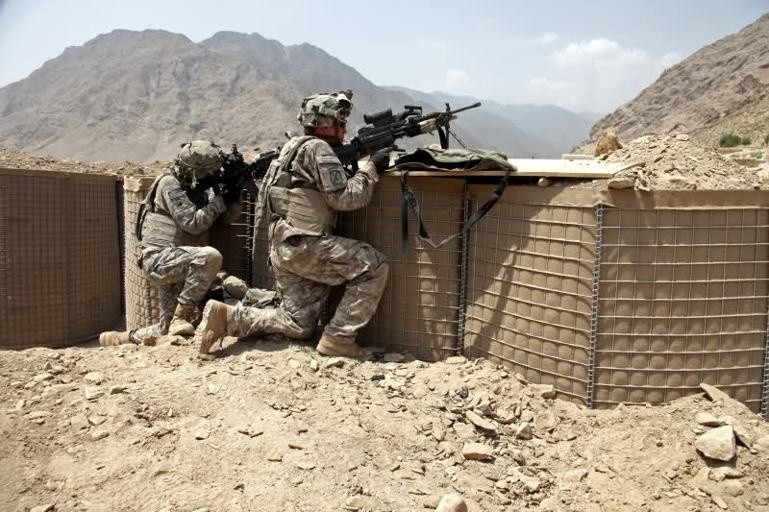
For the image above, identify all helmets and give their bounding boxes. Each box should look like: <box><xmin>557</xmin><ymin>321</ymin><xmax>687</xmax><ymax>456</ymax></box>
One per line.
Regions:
<box><xmin>296</xmin><ymin>90</ymin><xmax>356</xmax><ymax>131</ymax></box>
<box><xmin>176</xmin><ymin>139</ymin><xmax>226</xmax><ymax>180</ymax></box>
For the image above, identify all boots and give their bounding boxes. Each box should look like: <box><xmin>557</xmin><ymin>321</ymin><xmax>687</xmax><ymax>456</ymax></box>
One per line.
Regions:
<box><xmin>165</xmin><ymin>300</ymin><xmax>198</xmax><ymax>336</ymax></box>
<box><xmin>98</xmin><ymin>329</ymin><xmax>135</xmax><ymax>349</ymax></box>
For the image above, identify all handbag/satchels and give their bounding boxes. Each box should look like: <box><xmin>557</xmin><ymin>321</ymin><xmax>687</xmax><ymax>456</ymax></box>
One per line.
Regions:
<box><xmin>393</xmin><ymin>141</ymin><xmax>518</xmax><ymax>179</ymax></box>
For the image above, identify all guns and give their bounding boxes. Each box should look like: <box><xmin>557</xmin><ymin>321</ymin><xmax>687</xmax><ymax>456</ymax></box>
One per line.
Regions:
<box><xmin>192</xmin><ymin>144</ymin><xmax>282</xmax><ymax>196</ymax></box>
<box><xmin>336</xmin><ymin>101</ymin><xmax>481</xmax><ymax>172</ymax></box>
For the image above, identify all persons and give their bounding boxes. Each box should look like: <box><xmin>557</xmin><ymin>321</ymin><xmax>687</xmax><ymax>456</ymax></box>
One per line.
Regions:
<box><xmin>99</xmin><ymin>141</ymin><xmax>244</xmax><ymax>350</ymax></box>
<box><xmin>194</xmin><ymin>89</ymin><xmax>388</xmax><ymax>357</ymax></box>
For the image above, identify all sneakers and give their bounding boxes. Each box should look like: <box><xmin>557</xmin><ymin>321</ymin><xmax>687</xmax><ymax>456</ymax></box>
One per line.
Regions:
<box><xmin>315</xmin><ymin>329</ymin><xmax>377</xmax><ymax>362</ymax></box>
<box><xmin>191</xmin><ymin>297</ymin><xmax>230</xmax><ymax>356</ymax></box>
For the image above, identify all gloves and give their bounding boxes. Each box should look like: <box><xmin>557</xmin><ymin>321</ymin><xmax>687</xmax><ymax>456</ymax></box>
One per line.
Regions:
<box><xmin>369</xmin><ymin>145</ymin><xmax>395</xmax><ymax>177</ymax></box>
<box><xmin>218</xmin><ymin>179</ymin><xmax>245</xmax><ymax>209</ymax></box>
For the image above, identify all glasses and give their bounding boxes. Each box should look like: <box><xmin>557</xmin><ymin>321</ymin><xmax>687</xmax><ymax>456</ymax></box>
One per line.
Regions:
<box><xmin>335</xmin><ymin>119</ymin><xmax>348</xmax><ymax>130</ymax></box>
